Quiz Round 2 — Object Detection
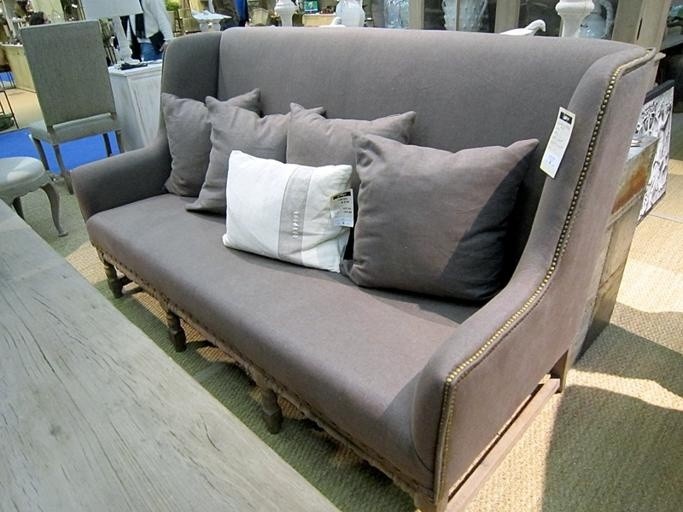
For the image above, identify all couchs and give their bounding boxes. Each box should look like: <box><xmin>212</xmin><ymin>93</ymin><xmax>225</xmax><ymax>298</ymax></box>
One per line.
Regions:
<box><xmin>66</xmin><ymin>23</ymin><xmax>667</xmax><ymax>509</ymax></box>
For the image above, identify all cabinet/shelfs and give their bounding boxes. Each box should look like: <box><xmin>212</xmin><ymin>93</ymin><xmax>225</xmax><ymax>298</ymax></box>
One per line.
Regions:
<box><xmin>106</xmin><ymin>59</ymin><xmax>163</xmax><ymax>155</ymax></box>
<box><xmin>575</xmin><ymin>135</ymin><xmax>658</xmax><ymax>366</ymax></box>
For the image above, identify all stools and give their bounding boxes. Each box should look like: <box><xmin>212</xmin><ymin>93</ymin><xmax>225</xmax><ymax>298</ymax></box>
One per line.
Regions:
<box><xmin>1</xmin><ymin>155</ymin><xmax>71</xmax><ymax>240</ymax></box>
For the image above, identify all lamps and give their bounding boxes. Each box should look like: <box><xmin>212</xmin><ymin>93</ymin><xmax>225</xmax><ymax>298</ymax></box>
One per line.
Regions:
<box><xmin>77</xmin><ymin>0</ymin><xmax>150</xmax><ymax>74</ymax></box>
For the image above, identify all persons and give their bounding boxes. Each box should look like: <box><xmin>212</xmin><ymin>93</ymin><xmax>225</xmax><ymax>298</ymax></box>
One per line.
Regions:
<box><xmin>127</xmin><ymin>0</ymin><xmax>175</xmax><ymax>61</ymax></box>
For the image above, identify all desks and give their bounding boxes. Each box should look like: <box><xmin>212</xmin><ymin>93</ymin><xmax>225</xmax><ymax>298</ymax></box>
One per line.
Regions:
<box><xmin>0</xmin><ymin>197</ymin><xmax>347</xmax><ymax>512</ymax></box>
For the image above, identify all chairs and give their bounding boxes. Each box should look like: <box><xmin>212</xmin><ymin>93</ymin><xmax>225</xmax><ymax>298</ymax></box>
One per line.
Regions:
<box><xmin>17</xmin><ymin>18</ymin><xmax>125</xmax><ymax>197</ymax></box>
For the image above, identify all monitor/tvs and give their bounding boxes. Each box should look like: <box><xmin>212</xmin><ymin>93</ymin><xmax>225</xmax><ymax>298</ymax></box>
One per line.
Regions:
<box><xmin>303</xmin><ymin>0</ymin><xmax>319</xmax><ymax>11</ymax></box>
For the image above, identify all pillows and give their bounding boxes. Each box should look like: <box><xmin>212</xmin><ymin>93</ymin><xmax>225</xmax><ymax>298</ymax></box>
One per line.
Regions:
<box><xmin>284</xmin><ymin>100</ymin><xmax>417</xmax><ymax>198</ymax></box>
<box><xmin>154</xmin><ymin>86</ymin><xmax>264</xmax><ymax>197</ymax></box>
<box><xmin>181</xmin><ymin>94</ymin><xmax>327</xmax><ymax>214</ymax></box>
<box><xmin>220</xmin><ymin>146</ymin><xmax>352</xmax><ymax>275</ymax></box>
<box><xmin>336</xmin><ymin>129</ymin><xmax>545</xmax><ymax>303</ymax></box>
